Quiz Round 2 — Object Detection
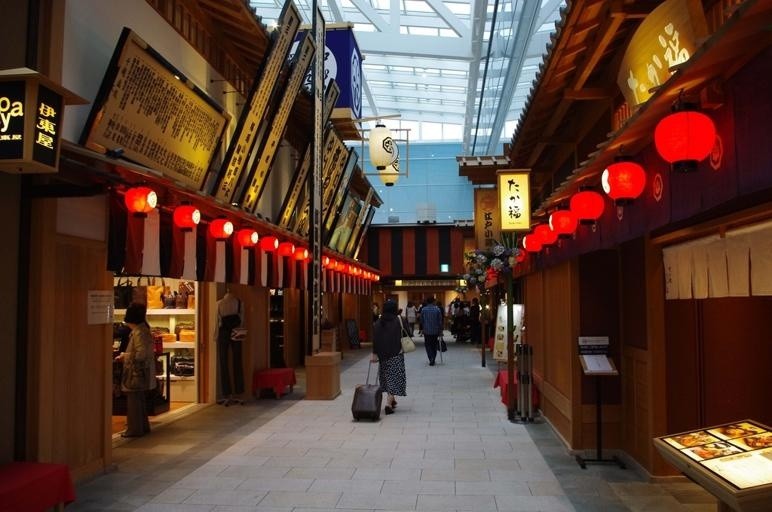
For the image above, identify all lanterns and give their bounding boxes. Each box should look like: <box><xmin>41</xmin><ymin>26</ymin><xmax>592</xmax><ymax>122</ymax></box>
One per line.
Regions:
<box><xmin>209</xmin><ymin>214</ymin><xmax>234</xmax><ymax>243</ymax></box>
<box><xmin>533</xmin><ymin>222</ymin><xmax>557</xmax><ymax>248</ymax></box>
<box><xmin>123</xmin><ymin>181</ymin><xmax>158</xmax><ymax>218</ymax></box>
<box><xmin>379</xmin><ymin>140</ymin><xmax>400</xmax><ymax>186</ymax></box>
<box><xmin>653</xmin><ymin>110</ymin><xmax>717</xmax><ymax>173</ymax></box>
<box><xmin>238</xmin><ymin>228</ymin><xmax>381</xmax><ymax>283</ymax></box>
<box><xmin>600</xmin><ymin>162</ymin><xmax>647</xmax><ymax>207</ymax></box>
<box><xmin>172</xmin><ymin>200</ymin><xmax>201</xmax><ymax>231</ymax></box>
<box><xmin>549</xmin><ymin>206</ymin><xmax>575</xmax><ymax>239</ymax></box>
<box><xmin>522</xmin><ymin>235</ymin><xmax>544</xmax><ymax>255</ymax></box>
<box><xmin>570</xmin><ymin>186</ymin><xmax>604</xmax><ymax>225</ymax></box>
<box><xmin>369</xmin><ymin>124</ymin><xmax>393</xmax><ymax>170</ymax></box>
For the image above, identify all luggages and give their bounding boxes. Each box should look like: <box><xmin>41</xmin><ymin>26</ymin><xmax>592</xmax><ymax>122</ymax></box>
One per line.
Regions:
<box><xmin>348</xmin><ymin>357</ymin><xmax>383</xmax><ymax>422</ymax></box>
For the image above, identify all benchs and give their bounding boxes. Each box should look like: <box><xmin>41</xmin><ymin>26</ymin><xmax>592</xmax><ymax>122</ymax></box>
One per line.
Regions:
<box><xmin>1</xmin><ymin>460</ymin><xmax>77</xmax><ymax>511</ymax></box>
<box><xmin>253</xmin><ymin>367</ymin><xmax>297</xmax><ymax>400</ymax></box>
<box><xmin>492</xmin><ymin>368</ymin><xmax>537</xmax><ymax>412</ymax></box>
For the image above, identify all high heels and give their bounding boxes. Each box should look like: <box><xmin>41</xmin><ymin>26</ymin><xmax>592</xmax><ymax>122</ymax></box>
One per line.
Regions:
<box><xmin>384</xmin><ymin>400</ymin><xmax>397</xmax><ymax>415</ymax></box>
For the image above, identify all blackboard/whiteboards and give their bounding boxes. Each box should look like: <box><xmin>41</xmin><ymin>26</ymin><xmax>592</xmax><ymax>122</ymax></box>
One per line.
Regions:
<box><xmin>345</xmin><ymin>319</ymin><xmax>360</xmax><ymax>346</ymax></box>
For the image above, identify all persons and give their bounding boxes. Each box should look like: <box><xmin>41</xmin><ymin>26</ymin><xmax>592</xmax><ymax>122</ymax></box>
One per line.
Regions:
<box><xmin>119</xmin><ymin>303</ymin><xmax>150</xmax><ymax>372</ymax></box>
<box><xmin>114</xmin><ymin>306</ymin><xmax>157</xmax><ymax>438</ymax></box>
<box><xmin>213</xmin><ymin>290</ymin><xmax>247</xmax><ymax>397</ymax></box>
<box><xmin>368</xmin><ymin>296</ymin><xmax>479</xmax><ymax>341</ymax></box>
<box><xmin>369</xmin><ymin>300</ymin><xmax>408</xmax><ymax>414</ymax></box>
<box><xmin>419</xmin><ymin>297</ymin><xmax>442</xmax><ymax>366</ymax></box>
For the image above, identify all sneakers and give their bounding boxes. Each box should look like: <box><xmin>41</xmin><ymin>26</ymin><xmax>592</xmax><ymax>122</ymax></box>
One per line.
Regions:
<box><xmin>121</xmin><ymin>428</ymin><xmax>152</xmax><ymax>437</ymax></box>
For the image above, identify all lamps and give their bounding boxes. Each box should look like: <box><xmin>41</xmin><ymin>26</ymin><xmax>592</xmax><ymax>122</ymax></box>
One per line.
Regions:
<box><xmin>335</xmin><ymin>113</ymin><xmax>403</xmax><ymax>171</ymax></box>
<box><xmin>341</xmin><ymin>128</ymin><xmax>410</xmax><ymax>186</ymax></box>
<box><xmin>124</xmin><ymin>182</ymin><xmax>379</xmax><ymax>283</ymax></box>
<box><xmin>514</xmin><ymin>103</ymin><xmax>715</xmax><ymax>265</ymax></box>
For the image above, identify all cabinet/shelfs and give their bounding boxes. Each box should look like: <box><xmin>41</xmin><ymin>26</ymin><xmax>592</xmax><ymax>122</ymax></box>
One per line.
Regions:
<box><xmin>111</xmin><ymin>307</ymin><xmax>195</xmax><ymax>403</ymax></box>
<box><xmin>322</xmin><ymin>327</ymin><xmax>341</xmax><ymax>352</ymax></box>
<box><xmin>113</xmin><ymin>351</ymin><xmax>171</xmax><ymax>417</ymax></box>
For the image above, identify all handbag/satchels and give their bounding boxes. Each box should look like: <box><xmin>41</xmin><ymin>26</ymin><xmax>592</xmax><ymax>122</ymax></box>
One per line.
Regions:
<box><xmin>146</xmin><ymin>276</ymin><xmax>170</xmax><ymax>310</ymax></box>
<box><xmin>175</xmin><ymin>281</ymin><xmax>194</xmax><ymax>309</ymax></box>
<box><xmin>400</xmin><ymin>328</ymin><xmax>416</xmax><ymax>353</ymax></box>
<box><xmin>113</xmin><ymin>276</ymin><xmax>135</xmax><ymax>310</ymax></box>
<box><xmin>122</xmin><ymin>350</ymin><xmax>152</xmax><ymax>390</ymax></box>
<box><xmin>437</xmin><ymin>333</ymin><xmax>448</xmax><ymax>352</ymax></box>
<box><xmin>112</xmin><ymin>321</ymin><xmax>132</xmax><ymax>340</ymax></box>
<box><xmin>230</xmin><ymin>326</ymin><xmax>248</xmax><ymax>341</ymax></box>
<box><xmin>132</xmin><ymin>275</ymin><xmax>152</xmax><ymax>309</ymax></box>
<box><xmin>161</xmin><ymin>290</ymin><xmax>176</xmax><ymax>309</ymax></box>
<box><xmin>170</xmin><ymin>347</ymin><xmax>195</xmax><ymax>376</ymax></box>
<box><xmin>187</xmin><ymin>288</ymin><xmax>196</xmax><ymax>310</ymax></box>
